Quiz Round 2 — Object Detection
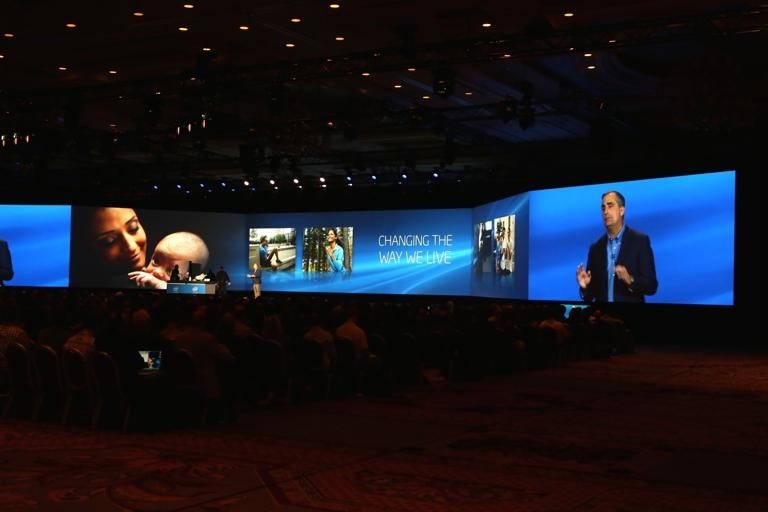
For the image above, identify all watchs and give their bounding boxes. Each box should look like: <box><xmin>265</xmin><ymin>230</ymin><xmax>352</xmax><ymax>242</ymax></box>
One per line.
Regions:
<box><xmin>630</xmin><ymin>277</ymin><xmax>635</xmax><ymax>282</ymax></box>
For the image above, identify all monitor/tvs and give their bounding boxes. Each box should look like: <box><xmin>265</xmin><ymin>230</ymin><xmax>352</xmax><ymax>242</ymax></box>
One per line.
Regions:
<box><xmin>138</xmin><ymin>350</ymin><xmax>163</xmax><ymax>370</ymax></box>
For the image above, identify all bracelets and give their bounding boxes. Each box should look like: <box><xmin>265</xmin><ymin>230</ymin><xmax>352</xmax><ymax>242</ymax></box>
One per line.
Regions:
<box><xmin>329</xmin><ymin>254</ymin><xmax>333</xmax><ymax>257</ymax></box>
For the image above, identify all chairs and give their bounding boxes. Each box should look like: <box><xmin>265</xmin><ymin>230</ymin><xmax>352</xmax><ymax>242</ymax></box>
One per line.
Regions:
<box><xmin>0</xmin><ymin>286</ymin><xmax>634</xmax><ymax>433</ymax></box>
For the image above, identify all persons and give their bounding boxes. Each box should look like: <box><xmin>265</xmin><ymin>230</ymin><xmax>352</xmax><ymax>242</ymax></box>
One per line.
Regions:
<box><xmin>170</xmin><ymin>264</ymin><xmax>179</xmax><ymax>280</ymax></box>
<box><xmin>504</xmin><ymin>227</ymin><xmax>513</xmax><ymax>271</ymax></box>
<box><xmin>216</xmin><ymin>265</ymin><xmax>231</xmax><ymax>304</ymax></box>
<box><xmin>324</xmin><ymin>228</ymin><xmax>346</xmax><ymax>272</ymax></box>
<box><xmin>496</xmin><ymin>236</ymin><xmax>504</xmax><ymax>271</ymax></box>
<box><xmin>259</xmin><ymin>235</ymin><xmax>282</xmax><ymax>267</ymax></box>
<box><xmin>78</xmin><ymin>207</ymin><xmax>149</xmax><ymax>289</ymax></box>
<box><xmin>501</xmin><ymin>227</ymin><xmax>507</xmax><ymax>259</ymax></box>
<box><xmin>2</xmin><ymin>286</ymin><xmax>335</xmax><ymax>421</ymax></box>
<box><xmin>328</xmin><ymin>300</ymin><xmax>629</xmax><ymax>395</ymax></box>
<box><xmin>127</xmin><ymin>231</ymin><xmax>209</xmax><ymax>290</ymax></box>
<box><xmin>576</xmin><ymin>190</ymin><xmax>659</xmax><ymax>302</ymax></box>
